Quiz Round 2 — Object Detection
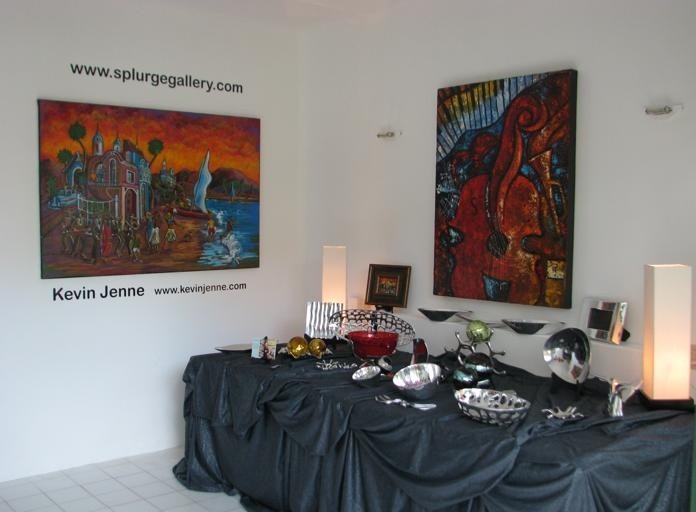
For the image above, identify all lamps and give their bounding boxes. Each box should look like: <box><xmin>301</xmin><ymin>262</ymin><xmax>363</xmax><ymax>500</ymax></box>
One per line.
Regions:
<box><xmin>640</xmin><ymin>263</ymin><xmax>695</xmax><ymax>403</ymax></box>
<box><xmin>642</xmin><ymin>103</ymin><xmax>682</xmax><ymax>121</ymax></box>
<box><xmin>376</xmin><ymin>125</ymin><xmax>405</xmax><ymax>141</ymax></box>
<box><xmin>322</xmin><ymin>245</ymin><xmax>349</xmax><ymax>314</ymax></box>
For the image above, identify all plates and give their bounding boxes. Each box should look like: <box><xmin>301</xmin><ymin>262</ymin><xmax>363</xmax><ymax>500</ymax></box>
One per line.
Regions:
<box><xmin>417</xmin><ymin>309</ymin><xmax>472</xmax><ymax>322</ymax></box>
<box><xmin>215</xmin><ymin>344</ymin><xmax>252</xmax><ymax>352</ymax></box>
<box><xmin>503</xmin><ymin>320</ymin><xmax>551</xmax><ymax>334</ymax></box>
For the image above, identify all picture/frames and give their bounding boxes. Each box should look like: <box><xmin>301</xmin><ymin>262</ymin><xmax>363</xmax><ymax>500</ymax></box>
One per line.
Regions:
<box><xmin>362</xmin><ymin>263</ymin><xmax>412</xmax><ymax>309</ymax></box>
<box><xmin>580</xmin><ymin>297</ymin><xmax>628</xmax><ymax>345</ymax></box>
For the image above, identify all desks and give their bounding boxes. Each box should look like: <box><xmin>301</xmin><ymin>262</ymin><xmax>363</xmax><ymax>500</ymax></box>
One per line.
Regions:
<box><xmin>169</xmin><ymin>337</ymin><xmax>695</xmax><ymax>511</ymax></box>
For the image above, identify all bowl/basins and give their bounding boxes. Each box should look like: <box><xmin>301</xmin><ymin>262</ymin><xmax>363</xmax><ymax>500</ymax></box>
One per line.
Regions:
<box><xmin>347</xmin><ymin>331</ymin><xmax>400</xmax><ymax>361</ymax></box>
<box><xmin>393</xmin><ymin>362</ymin><xmax>440</xmax><ymax>399</ymax></box>
<box><xmin>350</xmin><ymin>365</ymin><xmax>381</xmax><ymax>381</ymax></box>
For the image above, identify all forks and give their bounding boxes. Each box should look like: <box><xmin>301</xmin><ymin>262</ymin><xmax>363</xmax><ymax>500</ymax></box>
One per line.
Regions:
<box><xmin>375</xmin><ymin>393</ymin><xmax>437</xmax><ymax>412</ymax></box>
